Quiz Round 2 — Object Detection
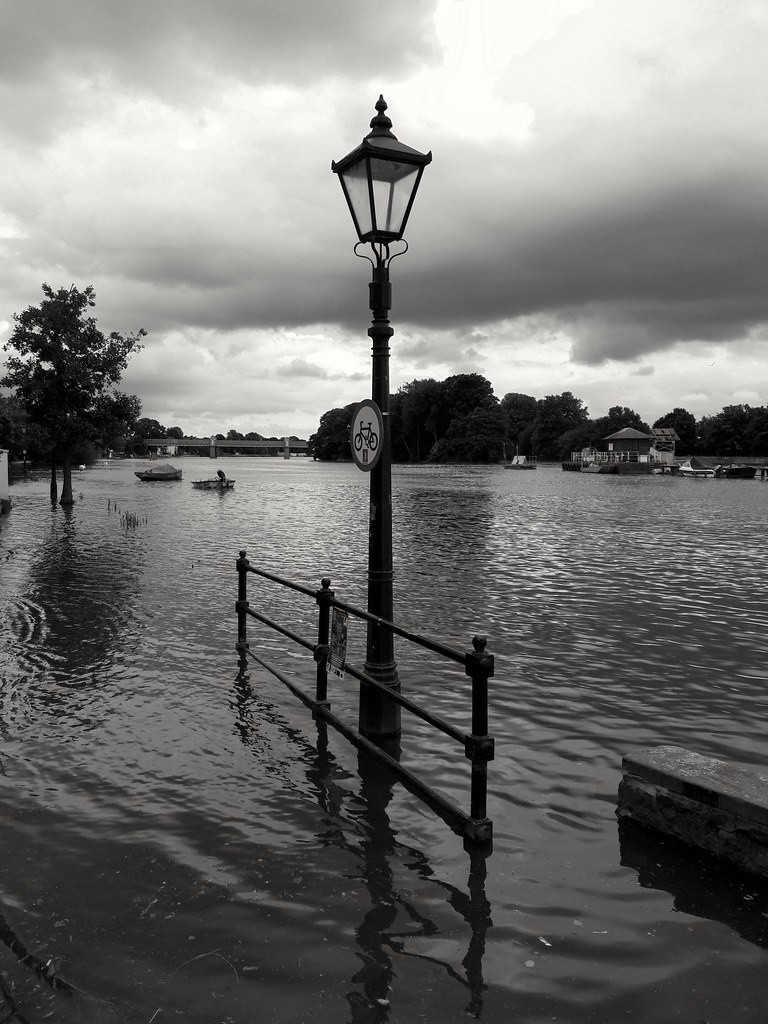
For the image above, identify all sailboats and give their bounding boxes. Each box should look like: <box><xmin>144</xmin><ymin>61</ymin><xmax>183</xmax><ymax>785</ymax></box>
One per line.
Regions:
<box><xmin>503</xmin><ymin>444</ymin><xmax>539</xmax><ymax>470</ymax></box>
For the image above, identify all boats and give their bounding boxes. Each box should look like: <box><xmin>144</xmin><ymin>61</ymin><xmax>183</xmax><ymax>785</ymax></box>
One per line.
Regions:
<box><xmin>713</xmin><ymin>464</ymin><xmax>758</xmax><ymax>479</ymax></box>
<box><xmin>191</xmin><ymin>477</ymin><xmax>236</xmax><ymax>489</ymax></box>
<box><xmin>134</xmin><ymin>462</ymin><xmax>182</xmax><ymax>482</ymax></box>
<box><xmin>678</xmin><ymin>458</ymin><xmax>713</xmax><ymax>479</ymax></box>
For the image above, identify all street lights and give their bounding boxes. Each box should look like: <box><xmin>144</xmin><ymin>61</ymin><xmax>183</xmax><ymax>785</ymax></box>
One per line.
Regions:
<box><xmin>328</xmin><ymin>90</ymin><xmax>433</xmax><ymax>769</ymax></box>
